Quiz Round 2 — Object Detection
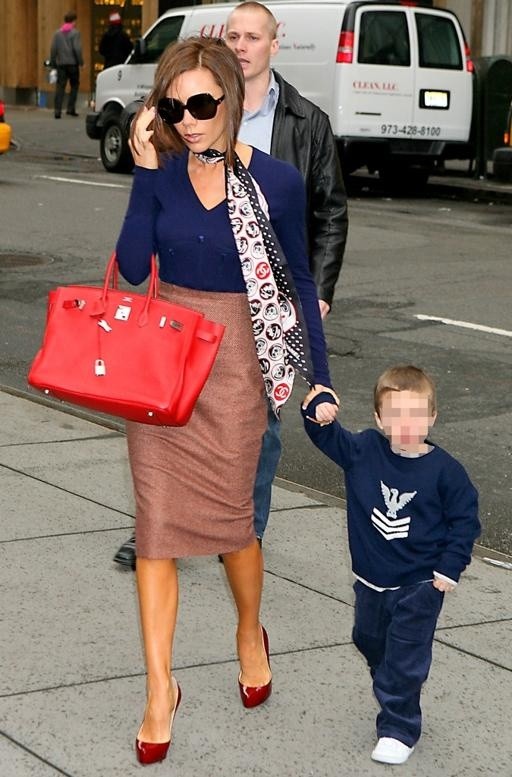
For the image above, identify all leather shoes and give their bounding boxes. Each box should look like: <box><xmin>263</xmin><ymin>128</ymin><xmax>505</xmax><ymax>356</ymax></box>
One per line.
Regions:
<box><xmin>114</xmin><ymin>535</ymin><xmax>136</xmax><ymax>566</ymax></box>
<box><xmin>56</xmin><ymin>111</ymin><xmax>78</xmax><ymax>118</ymax></box>
<box><xmin>238</xmin><ymin>625</ymin><xmax>273</xmax><ymax>708</ymax></box>
<box><xmin>135</xmin><ymin>677</ymin><xmax>181</xmax><ymax>764</ymax></box>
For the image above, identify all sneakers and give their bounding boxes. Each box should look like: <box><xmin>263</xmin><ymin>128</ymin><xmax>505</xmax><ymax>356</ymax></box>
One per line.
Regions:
<box><xmin>372</xmin><ymin>738</ymin><xmax>414</xmax><ymax>764</ymax></box>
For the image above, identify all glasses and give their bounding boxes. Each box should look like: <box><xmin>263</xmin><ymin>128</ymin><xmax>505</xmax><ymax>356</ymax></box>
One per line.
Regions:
<box><xmin>158</xmin><ymin>93</ymin><xmax>225</xmax><ymax>124</ymax></box>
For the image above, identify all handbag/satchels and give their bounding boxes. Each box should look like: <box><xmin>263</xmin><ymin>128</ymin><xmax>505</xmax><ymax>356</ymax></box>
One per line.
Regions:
<box><xmin>27</xmin><ymin>285</ymin><xmax>225</xmax><ymax>427</ymax></box>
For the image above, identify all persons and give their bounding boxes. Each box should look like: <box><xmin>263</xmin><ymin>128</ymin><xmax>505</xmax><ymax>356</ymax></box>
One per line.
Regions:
<box><xmin>295</xmin><ymin>363</ymin><xmax>483</xmax><ymax>770</ymax></box>
<box><xmin>98</xmin><ymin>12</ymin><xmax>134</xmax><ymax>71</ymax></box>
<box><xmin>50</xmin><ymin>11</ymin><xmax>84</xmax><ymax>121</ymax></box>
<box><xmin>115</xmin><ymin>1</ymin><xmax>353</xmax><ymax>584</ymax></box>
<box><xmin>110</xmin><ymin>35</ymin><xmax>340</xmax><ymax>766</ymax></box>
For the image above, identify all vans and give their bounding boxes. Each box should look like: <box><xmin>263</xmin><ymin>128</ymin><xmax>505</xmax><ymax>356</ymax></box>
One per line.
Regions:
<box><xmin>83</xmin><ymin>0</ymin><xmax>478</xmax><ymax>198</ymax></box>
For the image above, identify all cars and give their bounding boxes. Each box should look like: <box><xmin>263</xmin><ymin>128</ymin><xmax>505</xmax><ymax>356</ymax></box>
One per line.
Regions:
<box><xmin>0</xmin><ymin>98</ymin><xmax>12</xmax><ymax>157</ymax></box>
